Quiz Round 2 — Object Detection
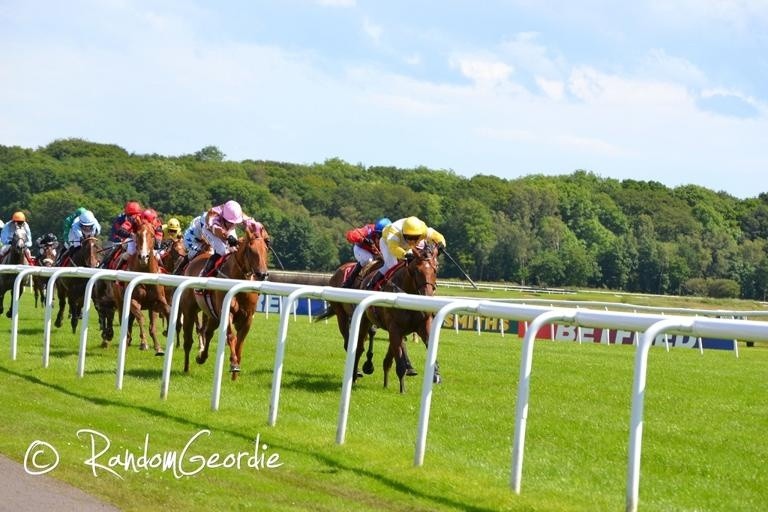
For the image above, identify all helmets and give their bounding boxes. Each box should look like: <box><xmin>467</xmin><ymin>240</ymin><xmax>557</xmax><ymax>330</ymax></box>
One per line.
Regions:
<box><xmin>374</xmin><ymin>218</ymin><xmax>392</xmax><ymax>233</ymax></box>
<box><xmin>75</xmin><ymin>207</ymin><xmax>95</xmax><ymax>226</ymax></box>
<box><xmin>125</xmin><ymin>201</ymin><xmax>142</xmax><ymax>216</ymax></box>
<box><xmin>402</xmin><ymin>216</ymin><xmax>426</xmax><ymax>236</ymax></box>
<box><xmin>168</xmin><ymin>218</ymin><xmax>180</xmax><ymax>231</ymax></box>
<box><xmin>222</xmin><ymin>200</ymin><xmax>244</xmax><ymax>224</ymax></box>
<box><xmin>12</xmin><ymin>211</ymin><xmax>26</xmax><ymax>222</ymax></box>
<box><xmin>141</xmin><ymin>208</ymin><xmax>159</xmax><ymax>223</ymax></box>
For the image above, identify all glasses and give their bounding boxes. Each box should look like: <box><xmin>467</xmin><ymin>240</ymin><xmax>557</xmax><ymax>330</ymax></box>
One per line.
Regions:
<box><xmin>404</xmin><ymin>235</ymin><xmax>419</xmax><ymax>242</ymax></box>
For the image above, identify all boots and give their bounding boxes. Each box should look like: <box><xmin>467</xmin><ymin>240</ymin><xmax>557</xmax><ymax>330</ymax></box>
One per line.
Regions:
<box><xmin>342</xmin><ymin>261</ymin><xmax>364</xmax><ymax>286</ymax></box>
<box><xmin>116</xmin><ymin>258</ymin><xmax>127</xmax><ymax>270</ymax></box>
<box><xmin>58</xmin><ymin>245</ymin><xmax>67</xmax><ymax>261</ymax></box>
<box><xmin>174</xmin><ymin>258</ymin><xmax>187</xmax><ymax>278</ymax></box>
<box><xmin>366</xmin><ymin>270</ymin><xmax>384</xmax><ymax>290</ymax></box>
<box><xmin>102</xmin><ymin>248</ymin><xmax>117</xmax><ymax>268</ymax></box>
<box><xmin>195</xmin><ymin>251</ymin><xmax>221</xmax><ymax>292</ymax></box>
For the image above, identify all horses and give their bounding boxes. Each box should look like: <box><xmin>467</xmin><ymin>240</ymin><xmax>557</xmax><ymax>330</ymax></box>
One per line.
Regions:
<box><xmin>313</xmin><ymin>262</ymin><xmax>417</xmax><ymax>377</ymax></box>
<box><xmin>0</xmin><ymin>223</ymin><xmax>31</xmax><ymax>318</ymax></box>
<box><xmin>180</xmin><ymin>225</ymin><xmax>271</xmax><ymax>381</ymax></box>
<box><xmin>350</xmin><ymin>240</ymin><xmax>442</xmax><ymax>395</ymax></box>
<box><xmin>33</xmin><ymin>215</ymin><xmax>209</xmax><ymax>360</ymax></box>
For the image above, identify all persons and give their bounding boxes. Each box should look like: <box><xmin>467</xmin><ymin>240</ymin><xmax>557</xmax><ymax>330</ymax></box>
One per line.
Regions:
<box><xmin>0</xmin><ymin>199</ymin><xmax>270</xmax><ymax>295</ymax></box>
<box><xmin>366</xmin><ymin>215</ymin><xmax>447</xmax><ymax>289</ymax></box>
<box><xmin>341</xmin><ymin>218</ymin><xmax>392</xmax><ymax>289</ymax></box>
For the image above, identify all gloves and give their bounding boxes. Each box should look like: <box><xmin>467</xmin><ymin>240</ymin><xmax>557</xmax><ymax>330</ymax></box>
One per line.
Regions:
<box><xmin>436</xmin><ymin>241</ymin><xmax>444</xmax><ymax>256</ymax></box>
<box><xmin>363</xmin><ymin>237</ymin><xmax>374</xmax><ymax>248</ymax></box>
<box><xmin>266</xmin><ymin>238</ymin><xmax>273</xmax><ymax>251</ymax></box>
<box><xmin>404</xmin><ymin>253</ymin><xmax>413</xmax><ymax>263</ymax></box>
<box><xmin>227</xmin><ymin>235</ymin><xmax>238</xmax><ymax>247</ymax></box>
